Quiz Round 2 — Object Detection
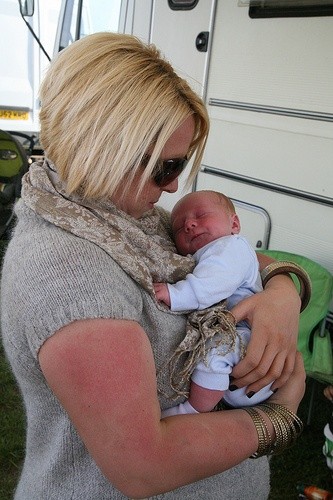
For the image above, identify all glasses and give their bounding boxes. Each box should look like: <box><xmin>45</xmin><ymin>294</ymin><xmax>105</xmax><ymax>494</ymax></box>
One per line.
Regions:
<box><xmin>141</xmin><ymin>143</ymin><xmax>199</xmax><ymax>188</ymax></box>
